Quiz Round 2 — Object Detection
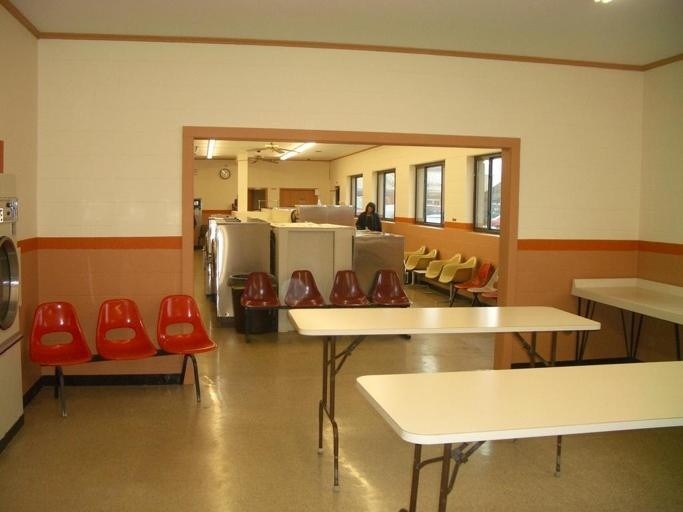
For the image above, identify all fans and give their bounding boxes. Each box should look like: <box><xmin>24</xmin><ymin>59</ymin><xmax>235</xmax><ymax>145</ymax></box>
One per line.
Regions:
<box><xmin>237</xmin><ymin>156</ymin><xmax>280</xmax><ymax>165</ymax></box>
<box><xmin>245</xmin><ymin>141</ymin><xmax>299</xmax><ymax>156</ymax></box>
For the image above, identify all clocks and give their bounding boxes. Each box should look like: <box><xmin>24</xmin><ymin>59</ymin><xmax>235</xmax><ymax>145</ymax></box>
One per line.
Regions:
<box><xmin>220</xmin><ymin>168</ymin><xmax>231</xmax><ymax>179</ymax></box>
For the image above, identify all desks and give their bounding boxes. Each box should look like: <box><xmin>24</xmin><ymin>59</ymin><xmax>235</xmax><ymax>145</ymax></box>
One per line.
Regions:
<box><xmin>340</xmin><ymin>360</ymin><xmax>682</xmax><ymax>507</ymax></box>
<box><xmin>570</xmin><ymin>276</ymin><xmax>683</xmax><ymax>361</ymax></box>
<box><xmin>285</xmin><ymin>305</ymin><xmax>602</xmax><ymax>492</ymax></box>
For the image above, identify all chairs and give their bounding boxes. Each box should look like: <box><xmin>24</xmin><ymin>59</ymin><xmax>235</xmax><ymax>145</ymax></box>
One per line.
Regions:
<box><xmin>240</xmin><ymin>268</ymin><xmax>414</xmax><ymax>342</ymax></box>
<box><xmin>394</xmin><ymin>245</ymin><xmax>500</xmax><ymax>302</ymax></box>
<box><xmin>31</xmin><ymin>293</ymin><xmax>217</xmax><ymax>418</ymax></box>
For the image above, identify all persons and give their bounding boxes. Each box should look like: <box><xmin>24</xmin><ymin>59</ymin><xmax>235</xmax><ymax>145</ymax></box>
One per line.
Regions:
<box><xmin>355</xmin><ymin>201</ymin><xmax>382</xmax><ymax>232</ymax></box>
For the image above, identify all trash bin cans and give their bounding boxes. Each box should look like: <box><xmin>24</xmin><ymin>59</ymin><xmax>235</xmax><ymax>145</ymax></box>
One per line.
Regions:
<box><xmin>228</xmin><ymin>272</ymin><xmax>277</xmax><ymax>335</ymax></box>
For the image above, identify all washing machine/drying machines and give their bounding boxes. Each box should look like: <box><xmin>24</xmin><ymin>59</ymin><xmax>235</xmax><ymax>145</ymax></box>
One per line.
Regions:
<box><xmin>0</xmin><ymin>171</ymin><xmax>25</xmax><ymax>453</ymax></box>
